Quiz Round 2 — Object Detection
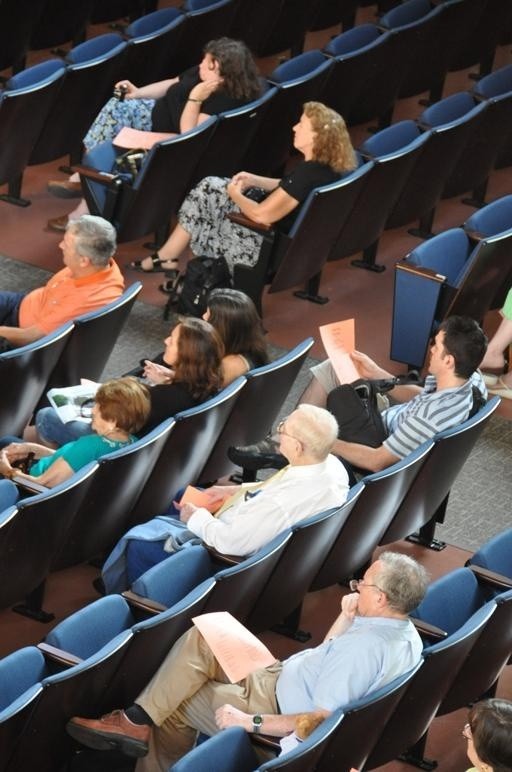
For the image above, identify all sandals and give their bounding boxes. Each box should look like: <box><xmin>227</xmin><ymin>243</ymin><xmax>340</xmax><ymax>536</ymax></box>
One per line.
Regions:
<box><xmin>126</xmin><ymin>252</ymin><xmax>180</xmax><ymax>272</ymax></box>
<box><xmin>158</xmin><ymin>276</ymin><xmax>181</xmax><ymax>294</ymax></box>
<box><xmin>228</xmin><ymin>435</ymin><xmax>289</xmax><ymax>470</ymax></box>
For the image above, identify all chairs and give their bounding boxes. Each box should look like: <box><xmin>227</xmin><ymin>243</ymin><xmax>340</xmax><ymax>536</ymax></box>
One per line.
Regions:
<box><xmin>1</xmin><ymin>505</ymin><xmax>50</xmax><ymax>627</ymax></box>
<box><xmin>0</xmin><ymin>460</ymin><xmax>100</xmax><ymax>624</ymax></box>
<box><xmin>34</xmin><ymin>281</ymin><xmax>144</xmax><ymax>414</ymax></box>
<box><xmin>192</xmin><ymin>79</ymin><xmax>281</xmax><ymax>189</ymax></box>
<box><xmin>197</xmin><ymin>337</ymin><xmax>315</xmax><ymax>489</ymax></box>
<box><xmin>460</xmin><ymin>194</ymin><xmax>512</xmax><ymax>245</ymax></box>
<box><xmin>167</xmin><ymin>707</ymin><xmax>344</xmax><ymax>772</ymax></box>
<box><xmin>496</xmin><ymin>0</ymin><xmax>512</xmax><ymax>75</ymax></box>
<box><xmin>306</xmin><ymin>0</ymin><xmax>356</xmax><ymax>33</ymax></box>
<box><xmin>360</xmin><ymin>120</ymin><xmax>436</xmax><ymax>240</ymax></box>
<box><xmin>70</xmin><ymin>114</ymin><xmax>219</xmax><ymax>251</ymax></box>
<box><xmin>36</xmin><ymin>575</ymin><xmax>216</xmax><ymax>718</ymax></box>
<box><xmin>0</xmin><ymin>0</ymin><xmax>26</xmax><ymax>76</ymax></box>
<box><xmin>320</xmin><ymin>5</ymin><xmax>446</xmax><ymax>107</ymax></box>
<box><xmin>308</xmin><ymin>438</ymin><xmax>435</xmax><ymax>593</ymax></box>
<box><xmin>65</xmin><ymin>15</ymin><xmax>187</xmax><ymax>88</ymax></box>
<box><xmin>468</xmin><ymin>63</ymin><xmax>512</xmax><ymax>170</ymax></box>
<box><xmin>0</xmin><ymin>628</ymin><xmax>134</xmax><ymax>772</ymax></box>
<box><xmin>376</xmin><ymin>0</ymin><xmax>496</xmax><ymax>81</ymax></box>
<box><xmin>5</xmin><ymin>41</ymin><xmax>128</xmax><ymax>176</ymax></box>
<box><xmin>125</xmin><ymin>1</ymin><xmax>236</xmax><ymax>76</ymax></box>
<box><xmin>248</xmin><ymin>654</ymin><xmax>424</xmax><ymax>772</ymax></box>
<box><xmin>121</xmin><ymin>527</ymin><xmax>293</xmax><ymax>627</ymax></box>
<box><xmin>0</xmin><ymin>682</ymin><xmax>43</xmax><ymax>772</ymax></box>
<box><xmin>122</xmin><ymin>376</ymin><xmax>247</xmax><ymax>537</ymax></box>
<box><xmin>26</xmin><ymin>0</ymin><xmax>86</xmax><ymax>52</ymax></box>
<box><xmin>408</xmin><ymin>563</ymin><xmax>512</xmax><ymax>718</ymax></box>
<box><xmin>86</xmin><ymin>1</ymin><xmax>157</xmax><ymax>25</ymax></box>
<box><xmin>0</xmin><ymin>59</ymin><xmax>69</xmax><ymax>207</ymax></box>
<box><xmin>227</xmin><ymin>161</ymin><xmax>374</xmax><ymax>305</ymax></box>
<box><xmin>181</xmin><ymin>0</ymin><xmax>306</xmax><ymax>70</ymax></box>
<box><xmin>0</xmin><ymin>320</ymin><xmax>75</xmax><ymax>439</ymax></box>
<box><xmin>200</xmin><ymin>481</ymin><xmax>365</xmax><ymax>644</ymax></box>
<box><xmin>267</xmin><ymin>50</ymin><xmax>392</xmax><ymax>134</ymax></box>
<box><xmin>341</xmin><ymin>130</ymin><xmax>432</xmax><ymax>272</ymax></box>
<box><xmin>12</xmin><ymin>417</ymin><xmax>176</xmax><ymax>569</ymax></box>
<box><xmin>390</xmin><ymin>227</ymin><xmax>512</xmax><ymax>387</ymax></box>
<box><xmin>378</xmin><ymin>396</ymin><xmax>501</xmax><ymax>552</ymax></box>
<box><xmin>415</xmin><ymin>93</ymin><xmax>494</xmax><ymax>209</ymax></box>
<box><xmin>470</xmin><ymin>527</ymin><xmax>512</xmax><ymax>579</ymax></box>
<box><xmin>361</xmin><ymin>599</ymin><xmax>497</xmax><ymax>772</ymax></box>
<box><xmin>357</xmin><ymin>0</ymin><xmax>403</xmax><ymax>18</ymax></box>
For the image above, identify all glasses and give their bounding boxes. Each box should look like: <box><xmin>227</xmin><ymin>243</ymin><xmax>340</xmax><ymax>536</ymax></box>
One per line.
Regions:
<box><xmin>462</xmin><ymin>720</ymin><xmax>472</xmax><ymax>741</ymax></box>
<box><xmin>276</xmin><ymin>416</ymin><xmax>299</xmax><ymax>442</ymax></box>
<box><xmin>350</xmin><ymin>580</ymin><xmax>381</xmax><ymax>594</ymax></box>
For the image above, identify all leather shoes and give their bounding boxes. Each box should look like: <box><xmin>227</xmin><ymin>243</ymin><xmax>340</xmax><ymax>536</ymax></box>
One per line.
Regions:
<box><xmin>48</xmin><ymin>214</ymin><xmax>69</xmax><ymax>232</ymax></box>
<box><xmin>66</xmin><ymin>710</ymin><xmax>151</xmax><ymax>758</ymax></box>
<box><xmin>46</xmin><ymin>178</ymin><xmax>84</xmax><ymax>200</ymax></box>
<box><xmin>92</xmin><ymin>576</ymin><xmax>107</xmax><ymax>597</ymax></box>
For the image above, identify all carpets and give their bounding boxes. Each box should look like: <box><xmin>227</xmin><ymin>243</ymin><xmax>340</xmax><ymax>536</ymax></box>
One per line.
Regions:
<box><xmin>1</xmin><ymin>254</ymin><xmax>512</xmax><ymax>554</ymax></box>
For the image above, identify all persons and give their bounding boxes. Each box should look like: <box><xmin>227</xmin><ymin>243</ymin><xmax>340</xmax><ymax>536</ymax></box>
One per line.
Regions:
<box><xmin>348</xmin><ymin>698</ymin><xmax>512</xmax><ymax>772</ymax></box>
<box><xmin>0</xmin><ymin>380</ymin><xmax>152</xmax><ymax>494</ymax></box>
<box><xmin>1</xmin><ymin>215</ymin><xmax>124</xmax><ymax>352</ymax></box>
<box><xmin>68</xmin><ymin>551</ymin><xmax>427</xmax><ymax>772</ymax></box>
<box><xmin>227</xmin><ymin>318</ymin><xmax>485</xmax><ymax>477</ymax></box>
<box><xmin>128</xmin><ymin>319</ymin><xmax>223</xmax><ymax>437</ymax></box>
<box><xmin>142</xmin><ymin>290</ymin><xmax>267</xmax><ymax>393</ymax></box>
<box><xmin>93</xmin><ymin>404</ymin><xmax>349</xmax><ymax>595</ymax></box>
<box><xmin>128</xmin><ymin>102</ymin><xmax>356</xmax><ymax>283</ymax></box>
<box><xmin>479</xmin><ymin>285</ymin><xmax>512</xmax><ymax>375</ymax></box>
<box><xmin>50</xmin><ymin>38</ymin><xmax>268</xmax><ymax>234</ymax></box>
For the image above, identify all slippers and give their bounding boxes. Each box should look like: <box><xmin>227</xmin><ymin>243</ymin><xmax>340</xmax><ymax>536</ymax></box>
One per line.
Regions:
<box><xmin>476</xmin><ymin>369</ymin><xmax>498</xmax><ymax>386</ymax></box>
<box><xmin>487</xmin><ymin>374</ymin><xmax>512</xmax><ymax>402</ymax></box>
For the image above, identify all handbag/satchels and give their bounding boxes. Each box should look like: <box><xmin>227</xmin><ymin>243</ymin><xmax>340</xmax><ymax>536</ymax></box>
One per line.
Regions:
<box><xmin>242</xmin><ymin>186</ymin><xmax>271</xmax><ymax>201</ymax></box>
<box><xmin>11</xmin><ymin>451</ymin><xmax>39</xmax><ymax>475</ymax></box>
